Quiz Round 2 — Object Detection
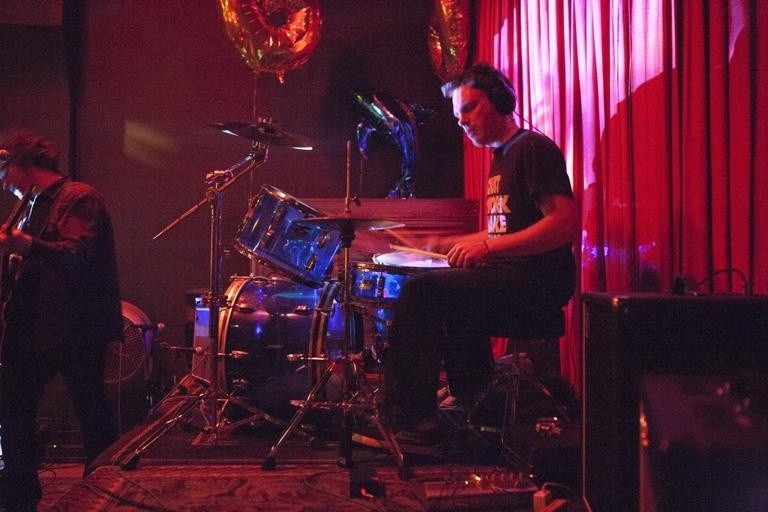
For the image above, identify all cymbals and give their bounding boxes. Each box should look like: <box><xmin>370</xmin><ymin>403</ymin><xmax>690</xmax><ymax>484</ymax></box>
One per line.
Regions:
<box><xmin>208</xmin><ymin>121</ymin><xmax>315</xmax><ymax>151</ymax></box>
<box><xmin>293</xmin><ymin>216</ymin><xmax>406</xmax><ymax>230</ymax></box>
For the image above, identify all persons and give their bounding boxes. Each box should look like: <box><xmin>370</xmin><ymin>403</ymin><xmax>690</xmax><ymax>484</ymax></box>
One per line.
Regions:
<box><xmin>0</xmin><ymin>124</ymin><xmax>124</xmax><ymax>512</ymax></box>
<box><xmin>345</xmin><ymin>60</ymin><xmax>581</xmax><ymax>457</ymax></box>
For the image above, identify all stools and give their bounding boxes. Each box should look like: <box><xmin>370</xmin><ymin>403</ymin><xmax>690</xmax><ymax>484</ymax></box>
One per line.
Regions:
<box><xmin>460</xmin><ymin>310</ymin><xmax>576</xmax><ymax>439</ymax></box>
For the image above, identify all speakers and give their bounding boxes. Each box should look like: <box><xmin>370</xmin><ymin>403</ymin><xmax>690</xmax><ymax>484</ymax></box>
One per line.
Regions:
<box><xmin>583</xmin><ymin>293</ymin><xmax>768</xmax><ymax>511</ymax></box>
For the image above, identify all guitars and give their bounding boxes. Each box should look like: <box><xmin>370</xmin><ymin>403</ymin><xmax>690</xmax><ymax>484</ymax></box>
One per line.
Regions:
<box><xmin>0</xmin><ymin>184</ymin><xmax>36</xmax><ymax>342</ymax></box>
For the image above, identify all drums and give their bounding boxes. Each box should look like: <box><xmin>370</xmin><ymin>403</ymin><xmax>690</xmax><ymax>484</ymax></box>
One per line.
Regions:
<box><xmin>372</xmin><ymin>251</ymin><xmax>453</xmax><ymax>273</ymax></box>
<box><xmin>350</xmin><ymin>259</ymin><xmax>418</xmax><ymax>309</ymax></box>
<box><xmin>231</xmin><ymin>185</ymin><xmax>343</xmax><ymax>288</ymax></box>
<box><xmin>214</xmin><ymin>276</ymin><xmax>363</xmax><ymax>434</ymax></box>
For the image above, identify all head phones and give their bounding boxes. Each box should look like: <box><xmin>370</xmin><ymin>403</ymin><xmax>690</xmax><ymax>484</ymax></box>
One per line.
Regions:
<box><xmin>489</xmin><ymin>82</ymin><xmax>519</xmax><ymax>114</ymax></box>
<box><xmin>0</xmin><ymin>149</ymin><xmax>17</xmax><ymax>165</ymax></box>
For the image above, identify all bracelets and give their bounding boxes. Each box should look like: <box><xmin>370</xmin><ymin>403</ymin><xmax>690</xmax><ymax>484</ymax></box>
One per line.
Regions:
<box><xmin>482</xmin><ymin>238</ymin><xmax>494</xmax><ymax>260</ymax></box>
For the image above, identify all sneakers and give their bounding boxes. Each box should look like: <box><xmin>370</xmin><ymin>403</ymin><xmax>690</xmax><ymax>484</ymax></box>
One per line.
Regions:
<box><xmin>351</xmin><ymin>420</ymin><xmax>440</xmax><ymax>456</ymax></box>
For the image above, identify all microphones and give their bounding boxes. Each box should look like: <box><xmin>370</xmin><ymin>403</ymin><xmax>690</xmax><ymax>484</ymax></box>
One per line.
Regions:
<box><xmin>411</xmin><ymin>103</ymin><xmax>438</xmax><ymax>124</ymax></box>
<box><xmin>131</xmin><ymin>322</ymin><xmax>166</xmax><ymax>333</ymax></box>
<box><xmin>162</xmin><ymin>345</ymin><xmax>204</xmax><ymax>355</ymax></box>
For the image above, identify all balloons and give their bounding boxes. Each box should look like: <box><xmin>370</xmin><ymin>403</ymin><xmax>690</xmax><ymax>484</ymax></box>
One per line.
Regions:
<box><xmin>218</xmin><ymin>0</ymin><xmax>326</xmax><ymax>85</ymax></box>
<box><xmin>346</xmin><ymin>83</ymin><xmax>440</xmax><ymax>201</ymax></box>
<box><xmin>426</xmin><ymin>1</ymin><xmax>473</xmax><ymax>86</ymax></box>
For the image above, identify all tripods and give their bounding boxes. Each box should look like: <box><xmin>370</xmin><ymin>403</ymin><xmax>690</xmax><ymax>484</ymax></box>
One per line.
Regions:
<box><xmin>262</xmin><ymin>148</ymin><xmax>406</xmax><ymax>470</ymax></box>
<box><xmin>119</xmin><ymin>158</ymin><xmax>322</xmax><ymax>468</ymax></box>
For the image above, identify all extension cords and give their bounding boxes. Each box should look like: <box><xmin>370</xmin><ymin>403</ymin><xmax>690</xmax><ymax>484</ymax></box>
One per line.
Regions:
<box><xmin>426</xmin><ymin>479</ymin><xmax>538</xmax><ymax>494</ymax></box>
